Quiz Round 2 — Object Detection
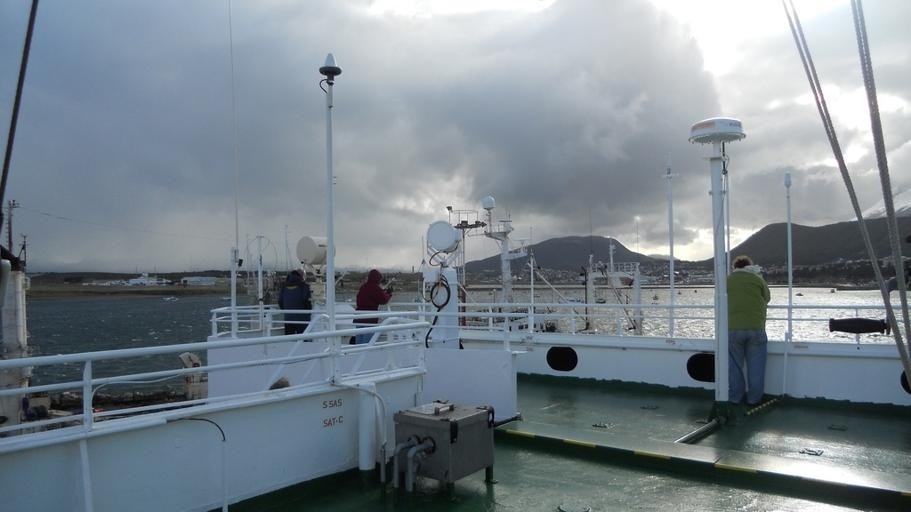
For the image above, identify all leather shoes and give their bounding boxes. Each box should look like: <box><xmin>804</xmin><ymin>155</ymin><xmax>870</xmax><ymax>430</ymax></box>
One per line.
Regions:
<box><xmin>747</xmin><ymin>402</ymin><xmax>761</xmax><ymax>409</ymax></box>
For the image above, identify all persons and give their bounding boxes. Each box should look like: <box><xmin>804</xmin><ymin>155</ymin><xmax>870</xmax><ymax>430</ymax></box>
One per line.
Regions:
<box><xmin>727</xmin><ymin>256</ymin><xmax>771</xmax><ymax>406</ymax></box>
<box><xmin>278</xmin><ymin>268</ymin><xmax>312</xmax><ymax>342</ymax></box>
<box><xmin>349</xmin><ymin>269</ymin><xmax>393</xmax><ymax>345</ymax></box>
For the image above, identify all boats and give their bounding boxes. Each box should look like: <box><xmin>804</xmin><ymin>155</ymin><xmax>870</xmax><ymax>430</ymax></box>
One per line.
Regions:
<box><xmin>558</xmin><ymin>287</ymin><xmax>661</xmax><ymax>305</ymax></box>
<box><xmin>465</xmin><ymin>194</ymin><xmax>558</xmax><ymax>332</ymax></box>
<box><xmin>128</xmin><ymin>272</ymin><xmax>173</xmax><ymax>286</ymax></box>
<box><xmin>575</xmin><ymin>261</ymin><xmax>634</xmax><ymax>285</ymax></box>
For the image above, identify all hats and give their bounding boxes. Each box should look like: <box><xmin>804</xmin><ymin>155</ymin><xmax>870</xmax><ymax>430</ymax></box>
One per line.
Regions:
<box><xmin>285</xmin><ymin>270</ymin><xmax>306</xmax><ymax>286</ymax></box>
